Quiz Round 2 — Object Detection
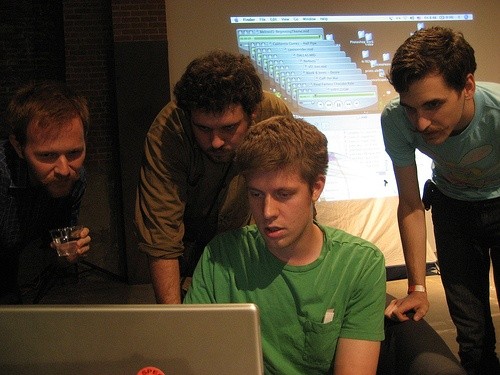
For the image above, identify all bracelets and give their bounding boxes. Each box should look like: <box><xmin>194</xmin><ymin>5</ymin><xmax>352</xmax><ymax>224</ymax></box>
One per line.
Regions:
<box><xmin>408</xmin><ymin>285</ymin><xmax>425</xmax><ymax>294</ymax></box>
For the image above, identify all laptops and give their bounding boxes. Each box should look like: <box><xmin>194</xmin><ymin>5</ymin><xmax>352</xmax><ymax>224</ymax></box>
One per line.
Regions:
<box><xmin>0</xmin><ymin>303</ymin><xmax>264</xmax><ymax>375</ymax></box>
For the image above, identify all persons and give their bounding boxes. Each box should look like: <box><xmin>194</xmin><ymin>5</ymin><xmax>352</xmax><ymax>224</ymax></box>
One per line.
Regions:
<box><xmin>132</xmin><ymin>51</ymin><xmax>294</xmax><ymax>304</ymax></box>
<box><xmin>182</xmin><ymin>115</ymin><xmax>386</xmax><ymax>375</ymax></box>
<box><xmin>381</xmin><ymin>26</ymin><xmax>500</xmax><ymax>375</ymax></box>
<box><xmin>0</xmin><ymin>79</ymin><xmax>91</xmax><ymax>305</ymax></box>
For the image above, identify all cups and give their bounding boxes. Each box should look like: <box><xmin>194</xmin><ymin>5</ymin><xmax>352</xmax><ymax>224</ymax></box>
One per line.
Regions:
<box><xmin>49</xmin><ymin>226</ymin><xmax>83</xmax><ymax>256</ymax></box>
